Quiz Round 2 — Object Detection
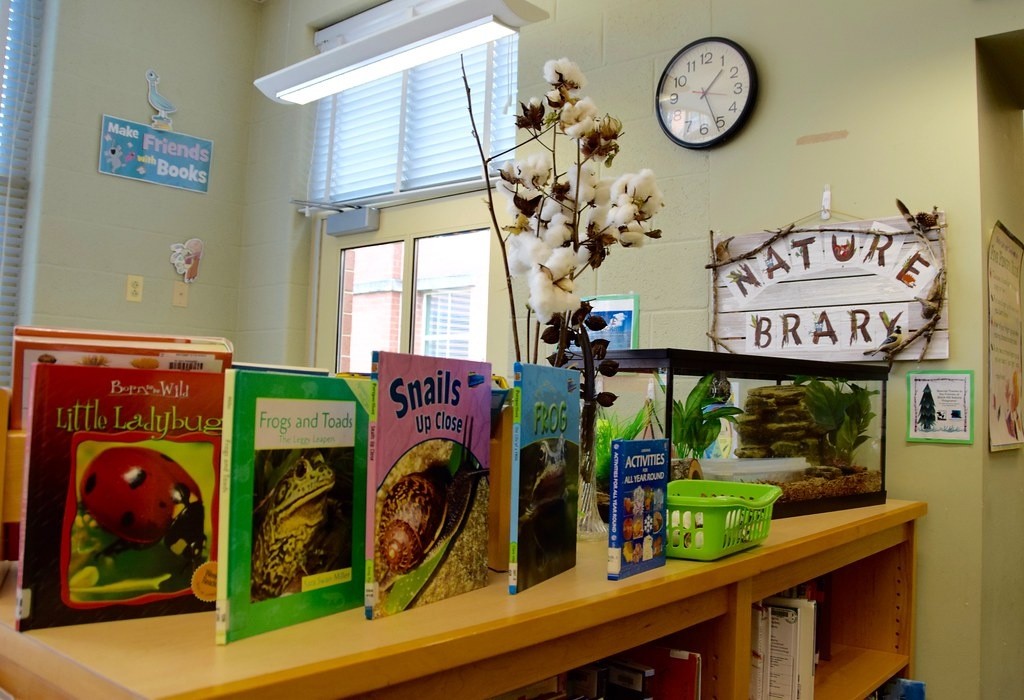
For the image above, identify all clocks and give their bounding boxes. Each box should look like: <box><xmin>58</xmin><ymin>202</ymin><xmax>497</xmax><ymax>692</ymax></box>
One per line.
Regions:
<box><xmin>655</xmin><ymin>36</ymin><xmax>757</xmax><ymax>150</ymax></box>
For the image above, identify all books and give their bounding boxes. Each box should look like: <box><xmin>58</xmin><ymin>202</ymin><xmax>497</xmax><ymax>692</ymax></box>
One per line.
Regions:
<box><xmin>508</xmin><ymin>362</ymin><xmax>580</xmax><ymax>594</ymax></box>
<box><xmin>7</xmin><ymin>322</ymin><xmax>511</xmax><ymax>647</ymax></box>
<box><xmin>606</xmin><ymin>439</ymin><xmax>667</xmax><ymax>580</ymax></box>
<box><xmin>510</xmin><ymin>576</ymin><xmax>832</xmax><ymax>700</ymax></box>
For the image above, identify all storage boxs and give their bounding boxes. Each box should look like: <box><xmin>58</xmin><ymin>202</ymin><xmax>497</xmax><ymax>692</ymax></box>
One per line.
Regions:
<box><xmin>662</xmin><ymin>478</ymin><xmax>785</xmax><ymax>560</ymax></box>
<box><xmin>559</xmin><ymin>347</ymin><xmax>887</xmax><ymax>524</ymax></box>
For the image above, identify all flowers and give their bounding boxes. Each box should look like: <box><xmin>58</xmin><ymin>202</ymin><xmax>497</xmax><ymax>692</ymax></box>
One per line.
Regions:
<box><xmin>461</xmin><ymin>50</ymin><xmax>667</xmax><ymax>417</ymax></box>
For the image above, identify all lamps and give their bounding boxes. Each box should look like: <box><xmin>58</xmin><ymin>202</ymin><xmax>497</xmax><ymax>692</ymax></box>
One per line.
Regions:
<box><xmin>252</xmin><ymin>12</ymin><xmax>522</xmax><ymax>109</ymax></box>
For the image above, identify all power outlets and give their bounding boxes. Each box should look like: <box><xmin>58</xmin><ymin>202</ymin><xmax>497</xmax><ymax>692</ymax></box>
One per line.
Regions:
<box><xmin>172</xmin><ymin>280</ymin><xmax>190</xmax><ymax>308</ymax></box>
<box><xmin>125</xmin><ymin>276</ymin><xmax>144</xmax><ymax>303</ymax></box>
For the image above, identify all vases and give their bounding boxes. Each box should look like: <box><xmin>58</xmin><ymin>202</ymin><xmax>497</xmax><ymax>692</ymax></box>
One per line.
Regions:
<box><xmin>578</xmin><ymin>417</ymin><xmax>609</xmax><ymax>538</ymax></box>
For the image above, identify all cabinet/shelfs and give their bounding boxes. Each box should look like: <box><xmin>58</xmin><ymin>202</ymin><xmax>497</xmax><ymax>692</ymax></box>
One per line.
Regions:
<box><xmin>60</xmin><ymin>497</ymin><xmax>928</xmax><ymax>700</ymax></box>
